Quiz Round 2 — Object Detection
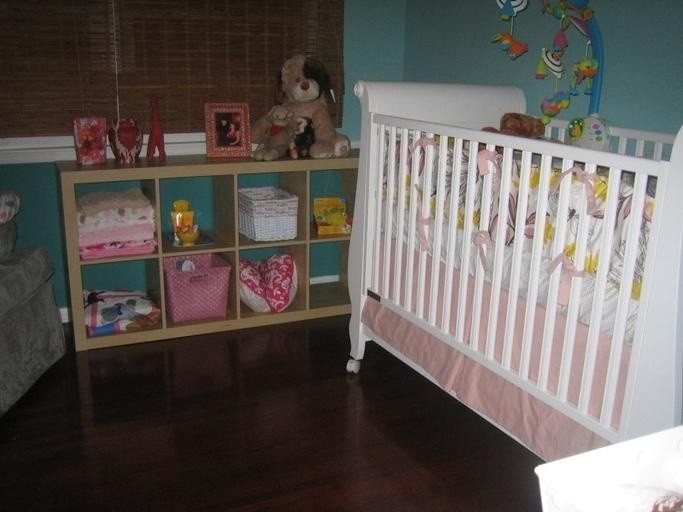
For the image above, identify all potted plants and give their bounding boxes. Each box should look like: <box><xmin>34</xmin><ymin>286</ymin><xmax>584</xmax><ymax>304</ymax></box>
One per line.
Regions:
<box><xmin>204</xmin><ymin>102</ymin><xmax>251</xmax><ymax>158</ymax></box>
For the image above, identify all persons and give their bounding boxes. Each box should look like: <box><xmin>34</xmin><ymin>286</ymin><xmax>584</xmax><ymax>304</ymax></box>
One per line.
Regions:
<box><xmin>216</xmin><ymin>119</ymin><xmax>228</xmax><ymax>145</ymax></box>
<box><xmin>224</xmin><ymin>122</ymin><xmax>241</xmax><ymax>147</ymax></box>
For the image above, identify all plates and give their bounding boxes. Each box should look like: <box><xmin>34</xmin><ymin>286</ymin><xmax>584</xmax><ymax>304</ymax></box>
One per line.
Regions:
<box><xmin>57</xmin><ymin>153</ymin><xmax>358</xmax><ymax>410</ymax></box>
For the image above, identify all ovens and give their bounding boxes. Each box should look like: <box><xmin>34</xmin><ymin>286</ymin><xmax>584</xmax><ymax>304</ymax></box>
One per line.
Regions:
<box><xmin>1</xmin><ymin>192</ymin><xmax>66</xmax><ymax>417</ymax></box>
<box><xmin>346</xmin><ymin>77</ymin><xmax>682</xmax><ymax>462</ymax></box>
